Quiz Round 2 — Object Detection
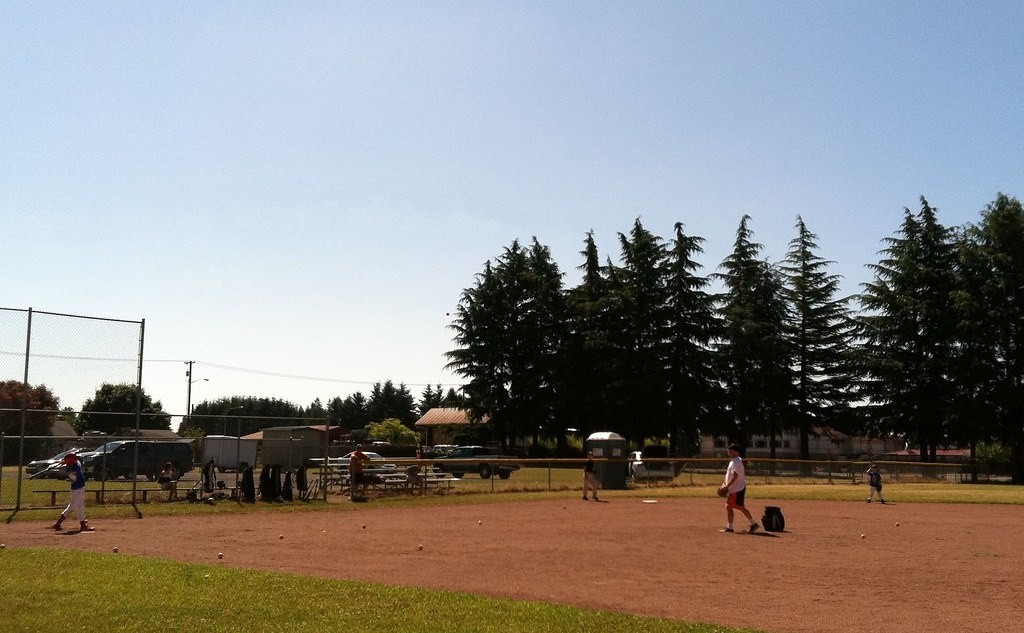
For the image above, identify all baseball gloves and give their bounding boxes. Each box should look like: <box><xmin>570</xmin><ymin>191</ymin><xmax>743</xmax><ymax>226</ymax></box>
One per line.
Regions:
<box><xmin>717</xmin><ymin>486</ymin><xmax>729</xmax><ymax>498</ymax></box>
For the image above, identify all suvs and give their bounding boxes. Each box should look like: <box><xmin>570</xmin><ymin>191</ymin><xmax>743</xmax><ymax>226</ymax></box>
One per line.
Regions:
<box><xmin>59</xmin><ymin>438</ymin><xmax>196</xmax><ymax>481</ymax></box>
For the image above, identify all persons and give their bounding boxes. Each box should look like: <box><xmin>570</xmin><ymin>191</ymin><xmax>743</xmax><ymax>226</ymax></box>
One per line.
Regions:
<box><xmin>582</xmin><ymin>451</ymin><xmax>598</xmax><ymax>500</ymax></box>
<box><xmin>717</xmin><ymin>443</ymin><xmax>760</xmax><ymax>534</ymax></box>
<box><xmin>866</xmin><ymin>465</ymin><xmax>885</xmax><ymax>504</ymax></box>
<box><xmin>349</xmin><ymin>444</ymin><xmax>370</xmax><ymax>501</ymax></box>
<box><xmin>157</xmin><ymin>463</ymin><xmax>177</xmax><ymax>501</ymax></box>
<box><xmin>50</xmin><ymin>454</ymin><xmax>95</xmax><ymax>532</ymax></box>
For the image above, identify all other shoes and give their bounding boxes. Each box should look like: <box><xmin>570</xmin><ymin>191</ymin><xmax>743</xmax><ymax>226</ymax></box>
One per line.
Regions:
<box><xmin>592</xmin><ymin>496</ymin><xmax>598</xmax><ymax>499</ymax></box>
<box><xmin>867</xmin><ymin>498</ymin><xmax>872</xmax><ymax>503</ymax></box>
<box><xmin>749</xmin><ymin>523</ymin><xmax>760</xmax><ymax>534</ymax></box>
<box><xmin>583</xmin><ymin>496</ymin><xmax>589</xmax><ymax>500</ymax></box>
<box><xmin>80</xmin><ymin>525</ymin><xmax>95</xmax><ymax>531</ymax></box>
<box><xmin>880</xmin><ymin>499</ymin><xmax>885</xmax><ymax>504</ymax></box>
<box><xmin>54</xmin><ymin>523</ymin><xmax>65</xmax><ymax>530</ymax></box>
<box><xmin>719</xmin><ymin>528</ymin><xmax>734</xmax><ymax>533</ymax></box>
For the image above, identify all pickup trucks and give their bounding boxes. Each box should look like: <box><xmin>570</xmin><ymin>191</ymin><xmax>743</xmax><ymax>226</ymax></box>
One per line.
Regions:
<box><xmin>432</xmin><ymin>446</ymin><xmax>520</xmax><ymax>480</ymax></box>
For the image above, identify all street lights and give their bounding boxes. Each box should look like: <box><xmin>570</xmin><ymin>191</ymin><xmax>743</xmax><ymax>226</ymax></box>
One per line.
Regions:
<box><xmin>220</xmin><ymin>406</ymin><xmax>244</xmax><ymax>471</ymax></box>
<box><xmin>186</xmin><ymin>378</ymin><xmax>209</xmax><ymax>424</ymax></box>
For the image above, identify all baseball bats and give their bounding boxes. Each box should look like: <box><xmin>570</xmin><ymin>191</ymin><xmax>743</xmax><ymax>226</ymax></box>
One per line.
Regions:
<box><xmin>29</xmin><ymin>462</ymin><xmax>63</xmax><ymax>479</ymax></box>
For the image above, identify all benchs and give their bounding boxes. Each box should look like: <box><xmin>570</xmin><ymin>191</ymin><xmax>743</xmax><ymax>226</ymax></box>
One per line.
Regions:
<box><xmin>312</xmin><ymin>464</ymin><xmax>461</xmax><ymax>496</ymax></box>
<box><xmin>33</xmin><ymin>486</ymin><xmax>260</xmax><ymax>506</ymax></box>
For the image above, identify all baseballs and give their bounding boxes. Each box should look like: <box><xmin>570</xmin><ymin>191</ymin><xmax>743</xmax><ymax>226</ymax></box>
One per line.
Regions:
<box><xmin>563</xmin><ymin>505</ymin><xmax>566</xmax><ymax>509</ymax></box>
<box><xmin>418</xmin><ymin>544</ymin><xmax>424</xmax><ymax>550</ymax></box>
<box><xmin>217</xmin><ymin>552</ymin><xmax>223</xmax><ymax>558</ymax></box>
<box><xmin>362</xmin><ymin>524</ymin><xmax>366</xmax><ymax>529</ymax></box>
<box><xmin>112</xmin><ymin>547</ymin><xmax>118</xmax><ymax>553</ymax></box>
<box><xmin>279</xmin><ymin>535</ymin><xmax>283</xmax><ymax>539</ymax></box>
<box><xmin>896</xmin><ymin>522</ymin><xmax>900</xmax><ymax>526</ymax></box>
<box><xmin>0</xmin><ymin>544</ymin><xmax>4</xmax><ymax>548</ymax></box>
<box><xmin>479</xmin><ymin>520</ymin><xmax>482</xmax><ymax>525</ymax></box>
<box><xmin>861</xmin><ymin>533</ymin><xmax>866</xmax><ymax>539</ymax></box>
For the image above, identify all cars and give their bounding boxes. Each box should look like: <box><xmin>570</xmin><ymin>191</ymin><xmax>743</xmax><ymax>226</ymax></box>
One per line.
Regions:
<box><xmin>337</xmin><ymin>452</ymin><xmax>395</xmax><ymax>475</ymax></box>
<box><xmin>629</xmin><ymin>442</ymin><xmax>687</xmax><ymax>480</ymax></box>
<box><xmin>372</xmin><ymin>441</ymin><xmax>392</xmax><ymax>447</ymax></box>
<box><xmin>25</xmin><ymin>448</ymin><xmax>77</xmax><ymax>476</ymax></box>
<box><xmin>51</xmin><ymin>452</ymin><xmax>88</xmax><ymax>482</ymax></box>
<box><xmin>433</xmin><ymin>445</ymin><xmax>461</xmax><ymax>455</ymax></box>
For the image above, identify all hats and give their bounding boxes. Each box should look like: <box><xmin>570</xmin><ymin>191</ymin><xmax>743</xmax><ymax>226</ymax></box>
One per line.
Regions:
<box><xmin>64</xmin><ymin>452</ymin><xmax>76</xmax><ymax>459</ymax></box>
<box><xmin>356</xmin><ymin>444</ymin><xmax>364</xmax><ymax>450</ymax></box>
<box><xmin>728</xmin><ymin>443</ymin><xmax>740</xmax><ymax>452</ymax></box>
